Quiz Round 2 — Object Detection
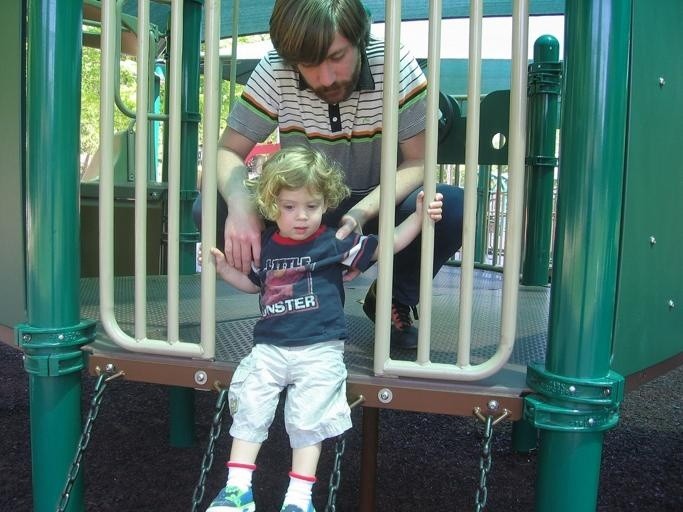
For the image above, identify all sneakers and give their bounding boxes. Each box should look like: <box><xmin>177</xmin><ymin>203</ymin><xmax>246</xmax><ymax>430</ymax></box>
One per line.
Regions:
<box><xmin>206</xmin><ymin>483</ymin><xmax>256</xmax><ymax>512</ymax></box>
<box><xmin>280</xmin><ymin>495</ymin><xmax>316</xmax><ymax>512</ymax></box>
<box><xmin>362</xmin><ymin>278</ymin><xmax>417</xmax><ymax>348</ymax></box>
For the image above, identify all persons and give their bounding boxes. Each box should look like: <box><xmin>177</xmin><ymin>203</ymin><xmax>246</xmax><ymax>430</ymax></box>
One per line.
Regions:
<box><xmin>193</xmin><ymin>1</ymin><xmax>470</xmax><ymax>349</ymax></box>
<box><xmin>195</xmin><ymin>146</ymin><xmax>444</xmax><ymax>512</ymax></box>
